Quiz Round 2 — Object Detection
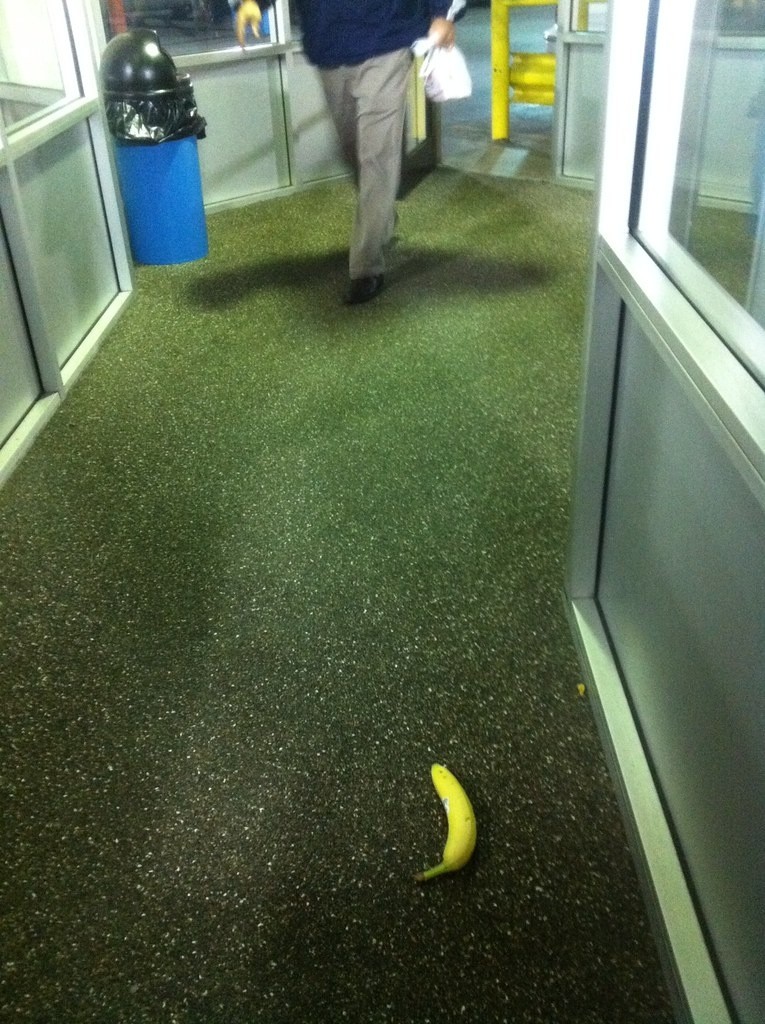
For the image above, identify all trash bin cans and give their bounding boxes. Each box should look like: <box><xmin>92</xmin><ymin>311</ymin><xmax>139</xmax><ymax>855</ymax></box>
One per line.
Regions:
<box><xmin>99</xmin><ymin>26</ymin><xmax>212</xmax><ymax>270</ymax></box>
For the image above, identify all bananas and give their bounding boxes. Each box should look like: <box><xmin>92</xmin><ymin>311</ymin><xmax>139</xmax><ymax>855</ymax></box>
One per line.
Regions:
<box><xmin>417</xmin><ymin>763</ymin><xmax>476</xmax><ymax>884</ymax></box>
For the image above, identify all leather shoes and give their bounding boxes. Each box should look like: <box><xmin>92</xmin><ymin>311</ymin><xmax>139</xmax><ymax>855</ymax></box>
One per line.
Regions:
<box><xmin>345</xmin><ymin>275</ymin><xmax>385</xmax><ymax>301</ymax></box>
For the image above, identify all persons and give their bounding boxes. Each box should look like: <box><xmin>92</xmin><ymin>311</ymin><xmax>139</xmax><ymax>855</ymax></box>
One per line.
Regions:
<box><xmin>234</xmin><ymin>0</ymin><xmax>469</xmax><ymax>307</ymax></box>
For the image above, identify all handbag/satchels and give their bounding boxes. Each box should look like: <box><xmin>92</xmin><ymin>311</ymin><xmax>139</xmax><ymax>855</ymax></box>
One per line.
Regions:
<box><xmin>412</xmin><ymin>31</ymin><xmax>472</xmax><ymax>102</ymax></box>
